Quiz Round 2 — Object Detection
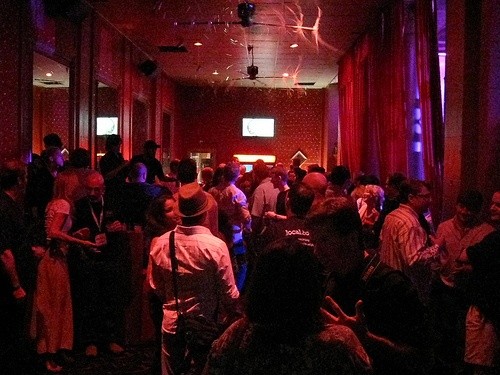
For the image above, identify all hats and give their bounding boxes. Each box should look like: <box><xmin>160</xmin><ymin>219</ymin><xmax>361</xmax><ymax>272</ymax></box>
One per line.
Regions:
<box><xmin>144</xmin><ymin>141</ymin><xmax>160</xmax><ymax>148</ymax></box>
<box><xmin>173</xmin><ymin>182</ymin><xmax>214</xmax><ymax>218</ymax></box>
<box><xmin>458</xmin><ymin>189</ymin><xmax>483</xmax><ymax>212</ymax></box>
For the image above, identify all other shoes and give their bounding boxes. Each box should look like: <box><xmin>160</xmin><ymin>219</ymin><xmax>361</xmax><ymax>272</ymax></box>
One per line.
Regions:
<box><xmin>109</xmin><ymin>342</ymin><xmax>124</xmax><ymax>352</ymax></box>
<box><xmin>45</xmin><ymin>360</ymin><xmax>62</xmax><ymax>371</ymax></box>
<box><xmin>86</xmin><ymin>345</ymin><xmax>98</xmax><ymax>356</ymax></box>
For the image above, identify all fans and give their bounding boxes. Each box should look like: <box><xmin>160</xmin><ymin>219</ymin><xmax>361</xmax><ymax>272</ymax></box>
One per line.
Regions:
<box><xmin>171</xmin><ymin>2</ymin><xmax>316</xmax><ymax>33</ymax></box>
<box><xmin>229</xmin><ymin>44</ymin><xmax>289</xmax><ymax>87</ymax></box>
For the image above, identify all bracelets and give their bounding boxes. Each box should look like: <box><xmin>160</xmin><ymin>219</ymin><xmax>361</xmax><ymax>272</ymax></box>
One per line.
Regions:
<box><xmin>12</xmin><ymin>283</ymin><xmax>22</xmax><ymax>292</ymax></box>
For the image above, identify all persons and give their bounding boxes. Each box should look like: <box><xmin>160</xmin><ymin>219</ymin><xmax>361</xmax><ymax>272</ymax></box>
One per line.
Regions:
<box><xmin>0</xmin><ymin>170</ymin><xmax>29</xmax><ymax>358</ymax></box>
<box><xmin>433</xmin><ymin>187</ymin><xmax>496</xmax><ymax>365</ymax></box>
<box><xmin>0</xmin><ymin>131</ymin><xmax>434</xmax><ymax>259</ymax></box>
<box><xmin>378</xmin><ymin>179</ymin><xmax>446</xmax><ymax>285</ymax></box>
<box><xmin>114</xmin><ymin>162</ymin><xmax>165</xmax><ymax>296</ymax></box>
<box><xmin>143</xmin><ymin>183</ymin><xmax>239</xmax><ymax>375</ymax></box>
<box><xmin>71</xmin><ymin>172</ymin><xmax>142</xmax><ymax>356</ymax></box>
<box><xmin>25</xmin><ymin>170</ymin><xmax>102</xmax><ymax>370</ymax></box>
<box><xmin>0</xmin><ymin>193</ymin><xmax>27</xmax><ymax>331</ymax></box>
<box><xmin>203</xmin><ymin>237</ymin><xmax>373</xmax><ymax>375</ymax></box>
<box><xmin>305</xmin><ymin>196</ymin><xmax>439</xmax><ymax>375</ymax></box>
<box><xmin>455</xmin><ymin>181</ymin><xmax>500</xmax><ymax>375</ymax></box>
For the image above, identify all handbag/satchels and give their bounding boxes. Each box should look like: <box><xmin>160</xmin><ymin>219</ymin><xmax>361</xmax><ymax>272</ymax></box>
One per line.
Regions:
<box><xmin>184</xmin><ymin>318</ymin><xmax>224</xmax><ymax>361</ymax></box>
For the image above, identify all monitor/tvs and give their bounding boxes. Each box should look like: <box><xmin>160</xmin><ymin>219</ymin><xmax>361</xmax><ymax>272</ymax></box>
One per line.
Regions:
<box><xmin>240</xmin><ymin>115</ymin><xmax>277</xmax><ymax>139</ymax></box>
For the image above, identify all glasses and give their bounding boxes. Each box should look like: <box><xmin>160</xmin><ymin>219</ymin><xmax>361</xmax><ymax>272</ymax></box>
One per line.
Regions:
<box><xmin>418</xmin><ymin>192</ymin><xmax>432</xmax><ymax>199</ymax></box>
